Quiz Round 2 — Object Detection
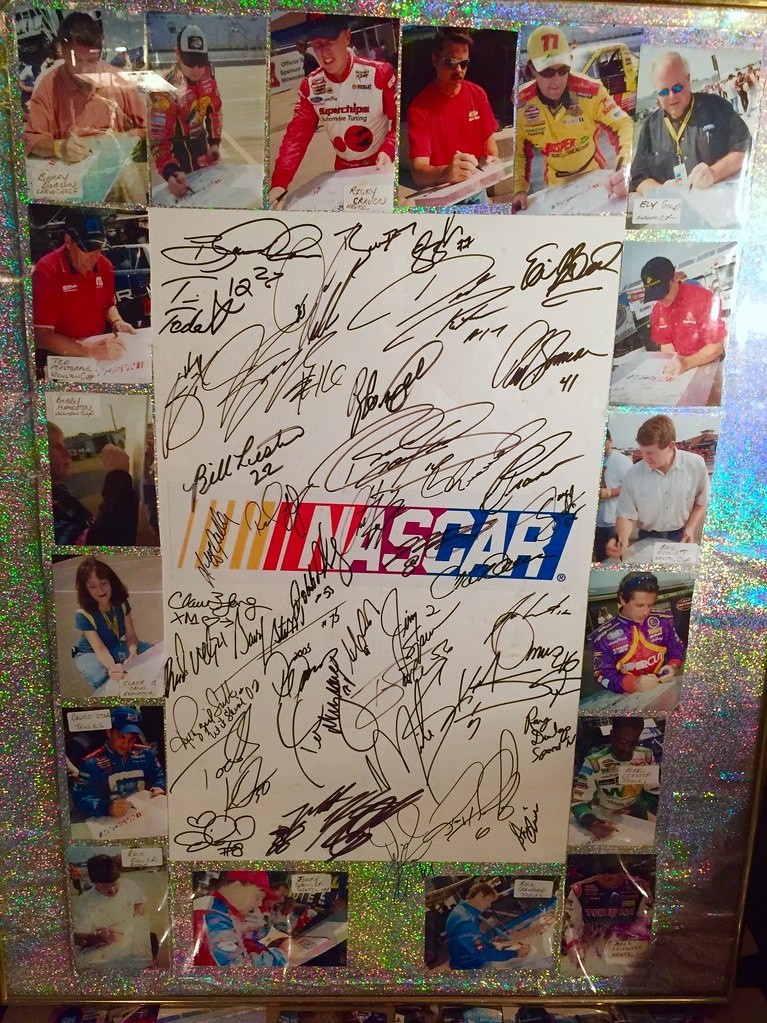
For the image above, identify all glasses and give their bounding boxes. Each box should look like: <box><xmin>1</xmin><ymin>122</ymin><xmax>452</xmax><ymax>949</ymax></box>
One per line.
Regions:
<box><xmin>623</xmin><ymin>574</ymin><xmax>658</xmax><ymax>593</ymax></box>
<box><xmin>437</xmin><ymin>54</ymin><xmax>470</xmax><ymax>70</ymax></box>
<box><xmin>75</xmin><ymin>241</ymin><xmax>104</xmax><ymax>253</ymax></box>
<box><xmin>655</xmin><ymin>76</ymin><xmax>688</xmax><ymax>97</ymax></box>
<box><xmin>533</xmin><ymin>65</ymin><xmax>571</xmax><ymax>78</ymax></box>
<box><xmin>181</xmin><ymin>59</ymin><xmax>206</xmax><ymax>68</ymax></box>
<box><xmin>594</xmin><ymin>866</ymin><xmax>622</xmax><ymax>875</ymax></box>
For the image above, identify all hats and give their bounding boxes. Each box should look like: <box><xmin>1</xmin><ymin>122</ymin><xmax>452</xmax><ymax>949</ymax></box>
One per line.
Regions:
<box><xmin>64</xmin><ymin>207</ymin><xmax>106</xmax><ymax>244</ymax></box>
<box><xmin>219</xmin><ymin>870</ymin><xmax>282</xmax><ymax>902</ymax></box>
<box><xmin>87</xmin><ymin>854</ymin><xmax>121</xmax><ymax>884</ymax></box>
<box><xmin>177</xmin><ymin>24</ymin><xmax>209</xmax><ymax>65</ymax></box>
<box><xmin>641</xmin><ymin>257</ymin><xmax>675</xmax><ymax>304</ymax></box>
<box><xmin>304</xmin><ymin>13</ymin><xmax>349</xmax><ymax>42</ymax></box>
<box><xmin>527</xmin><ymin>25</ymin><xmax>571</xmax><ymax>72</ymax></box>
<box><xmin>109</xmin><ymin>706</ymin><xmax>145</xmax><ymax>735</ymax></box>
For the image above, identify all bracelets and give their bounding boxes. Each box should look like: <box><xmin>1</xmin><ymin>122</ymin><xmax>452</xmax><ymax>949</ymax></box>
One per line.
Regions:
<box><xmin>607</xmin><ymin>488</ymin><xmax>611</xmax><ymax>497</ymax></box>
<box><xmin>54</xmin><ymin>139</ymin><xmax>63</xmax><ymax>160</ymax></box>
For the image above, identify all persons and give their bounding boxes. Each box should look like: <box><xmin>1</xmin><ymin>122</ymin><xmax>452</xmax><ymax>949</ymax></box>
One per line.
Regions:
<box><xmin>268</xmin><ymin>14</ymin><xmax>396</xmax><ymax>212</ymax></box>
<box><xmin>72</xmin><ymin>707</ymin><xmax>165</xmax><ymax>818</ymax></box>
<box><xmin>33</xmin><ymin>208</ymin><xmax>138</xmax><ymax>362</ymax></box>
<box><xmin>605</xmin><ymin>415</ymin><xmax>710</xmax><ymax>559</ymax></box>
<box><xmin>562</xmin><ymin>855</ymin><xmax>654</xmax><ymax>968</ymax></box>
<box><xmin>152</xmin><ymin>22</ymin><xmax>224</xmax><ymax>197</ymax></box>
<box><xmin>594</xmin><ymin>429</ymin><xmax>633</xmax><ymax>563</ymax></box>
<box><xmin>628</xmin><ymin>51</ymin><xmax>752</xmax><ymax>200</ymax></box>
<box><xmin>23</xmin><ymin>10</ymin><xmax>147</xmax><ymax>205</ymax></box>
<box><xmin>72</xmin><ymin>556</ymin><xmax>154</xmax><ymax>689</ymax></box>
<box><xmin>571</xmin><ymin>717</ymin><xmax>660</xmax><ymax>839</ymax></box>
<box><xmin>47</xmin><ymin>421</ymin><xmax>138</xmax><ymax>548</ymax></box>
<box><xmin>73</xmin><ymin>854</ymin><xmax>146</xmax><ymax>969</ymax></box>
<box><xmin>586</xmin><ymin>572</ymin><xmax>684</xmax><ymax>695</ymax></box>
<box><xmin>444</xmin><ymin>883</ymin><xmax>530</xmax><ymax>971</ymax></box>
<box><xmin>193</xmin><ymin>871</ymin><xmax>317</xmax><ymax>966</ymax></box>
<box><xmin>511</xmin><ymin>26</ymin><xmax>632</xmax><ymax>215</ymax></box>
<box><xmin>641</xmin><ymin>256</ymin><xmax>726</xmax><ymax>372</ymax></box>
<box><xmin>407</xmin><ymin>28</ymin><xmax>498</xmax><ymax>205</ymax></box>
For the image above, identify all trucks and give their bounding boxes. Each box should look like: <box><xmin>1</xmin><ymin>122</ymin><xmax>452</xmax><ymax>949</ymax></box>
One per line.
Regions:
<box><xmin>632</xmin><ymin>442</ymin><xmax>713</xmax><ymax>464</ymax></box>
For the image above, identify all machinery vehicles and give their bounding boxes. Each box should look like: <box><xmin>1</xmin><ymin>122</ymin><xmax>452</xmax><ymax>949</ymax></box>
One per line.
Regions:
<box><xmin>573</xmin><ymin>42</ymin><xmax>640</xmax><ymax>112</ymax></box>
